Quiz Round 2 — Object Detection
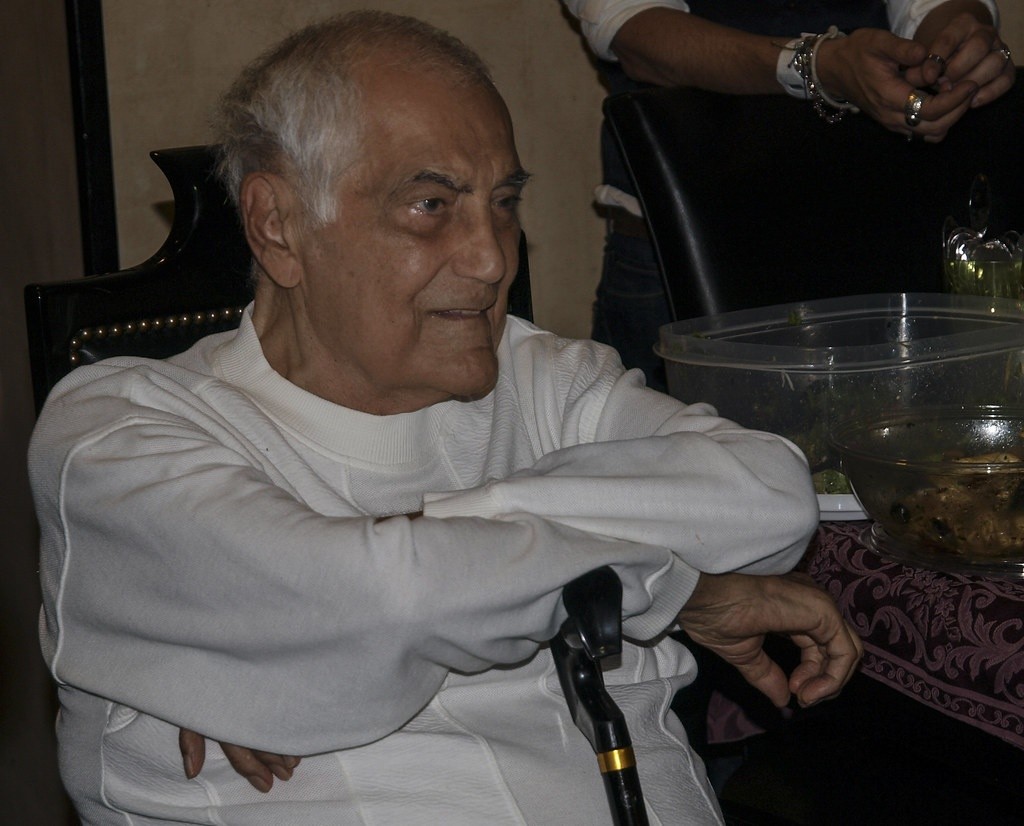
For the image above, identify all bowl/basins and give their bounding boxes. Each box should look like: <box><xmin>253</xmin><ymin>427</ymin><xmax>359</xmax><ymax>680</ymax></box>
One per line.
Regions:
<box><xmin>944</xmin><ymin>258</ymin><xmax>1024</xmax><ymax>300</ymax></box>
<box><xmin>829</xmin><ymin>402</ymin><xmax>1024</xmax><ymax>571</ymax></box>
<box><xmin>652</xmin><ymin>292</ymin><xmax>1024</xmax><ymax>495</ymax></box>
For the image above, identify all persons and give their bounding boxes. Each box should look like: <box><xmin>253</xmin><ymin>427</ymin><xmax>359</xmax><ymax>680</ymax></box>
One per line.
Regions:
<box><xmin>562</xmin><ymin>0</ymin><xmax>1023</xmax><ymax>391</ymax></box>
<box><xmin>26</xmin><ymin>10</ymin><xmax>860</xmax><ymax>825</ymax></box>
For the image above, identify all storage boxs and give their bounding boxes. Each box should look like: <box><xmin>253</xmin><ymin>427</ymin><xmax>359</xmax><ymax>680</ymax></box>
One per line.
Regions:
<box><xmin>653</xmin><ymin>293</ymin><xmax>1024</xmax><ymax>494</ymax></box>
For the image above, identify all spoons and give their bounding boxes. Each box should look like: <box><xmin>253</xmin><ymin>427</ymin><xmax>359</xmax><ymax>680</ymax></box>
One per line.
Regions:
<box><xmin>942</xmin><ymin>175</ymin><xmax>1024</xmax><ymax>258</ymax></box>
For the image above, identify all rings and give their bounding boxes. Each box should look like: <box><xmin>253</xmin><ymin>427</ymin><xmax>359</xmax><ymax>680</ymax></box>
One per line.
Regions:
<box><xmin>903</xmin><ymin>89</ymin><xmax>929</xmax><ymax>127</ymax></box>
<box><xmin>997</xmin><ymin>43</ymin><xmax>1012</xmax><ymax>63</ymax></box>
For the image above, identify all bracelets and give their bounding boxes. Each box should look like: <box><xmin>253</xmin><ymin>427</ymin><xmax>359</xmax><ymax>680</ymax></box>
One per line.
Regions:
<box><xmin>774</xmin><ymin>26</ymin><xmax>859</xmax><ymax>125</ymax></box>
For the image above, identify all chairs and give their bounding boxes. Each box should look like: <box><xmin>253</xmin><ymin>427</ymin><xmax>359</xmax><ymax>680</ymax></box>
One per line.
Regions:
<box><xmin>23</xmin><ymin>144</ymin><xmax>534</xmax><ymax>420</ymax></box>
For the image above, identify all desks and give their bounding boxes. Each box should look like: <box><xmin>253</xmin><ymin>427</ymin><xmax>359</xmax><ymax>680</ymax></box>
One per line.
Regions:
<box><xmin>707</xmin><ymin>521</ymin><xmax>1023</xmax><ymax>826</ymax></box>
